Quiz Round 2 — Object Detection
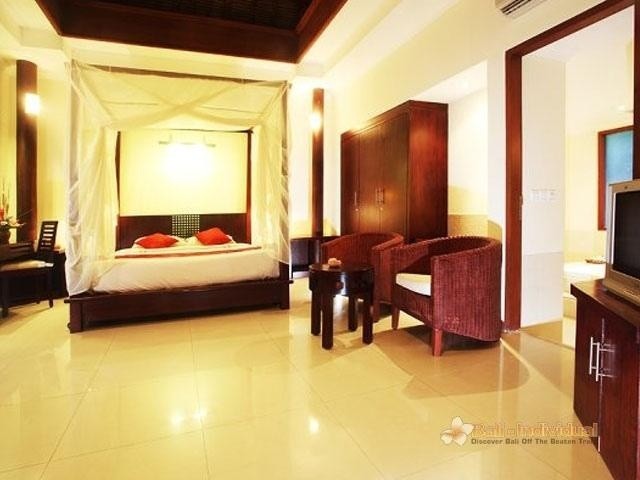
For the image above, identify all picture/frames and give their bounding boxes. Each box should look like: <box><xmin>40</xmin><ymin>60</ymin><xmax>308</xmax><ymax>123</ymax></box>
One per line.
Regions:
<box><xmin>307</xmin><ymin>260</ymin><xmax>374</xmax><ymax>350</ymax></box>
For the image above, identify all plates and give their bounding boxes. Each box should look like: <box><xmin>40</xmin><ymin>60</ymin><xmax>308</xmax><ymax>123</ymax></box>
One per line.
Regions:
<box><xmin>338</xmin><ymin>100</ymin><xmax>447</xmax><ymax>243</ymax></box>
<box><xmin>567</xmin><ymin>277</ymin><xmax>638</xmax><ymax>480</ymax></box>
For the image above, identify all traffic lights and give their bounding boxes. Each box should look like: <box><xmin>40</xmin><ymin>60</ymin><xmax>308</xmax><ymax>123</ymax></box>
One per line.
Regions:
<box><xmin>132</xmin><ymin>224</ymin><xmax>238</xmax><ymax>252</ymax></box>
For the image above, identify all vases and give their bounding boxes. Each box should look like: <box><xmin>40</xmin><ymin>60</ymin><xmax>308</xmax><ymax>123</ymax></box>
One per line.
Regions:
<box><xmin>0</xmin><ymin>177</ymin><xmax>33</xmax><ymax>230</ymax></box>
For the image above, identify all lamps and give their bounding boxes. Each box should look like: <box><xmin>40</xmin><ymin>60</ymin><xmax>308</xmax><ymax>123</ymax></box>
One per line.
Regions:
<box><xmin>317</xmin><ymin>229</ymin><xmax>504</xmax><ymax>357</ymax></box>
<box><xmin>0</xmin><ymin>219</ymin><xmax>59</xmax><ymax>318</ymax></box>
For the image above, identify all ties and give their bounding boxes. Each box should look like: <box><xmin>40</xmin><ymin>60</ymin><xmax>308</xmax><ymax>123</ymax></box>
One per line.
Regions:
<box><xmin>602</xmin><ymin>178</ymin><xmax>640</xmax><ymax>307</ymax></box>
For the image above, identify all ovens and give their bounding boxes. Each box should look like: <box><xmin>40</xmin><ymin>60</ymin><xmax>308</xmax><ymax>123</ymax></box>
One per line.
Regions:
<box><xmin>62</xmin><ymin>213</ymin><xmax>293</xmax><ymax>335</ymax></box>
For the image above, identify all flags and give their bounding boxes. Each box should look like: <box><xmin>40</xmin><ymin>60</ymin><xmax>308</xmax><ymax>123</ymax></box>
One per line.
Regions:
<box><xmin>1</xmin><ymin>224</ymin><xmax>12</xmax><ymax>245</ymax></box>
